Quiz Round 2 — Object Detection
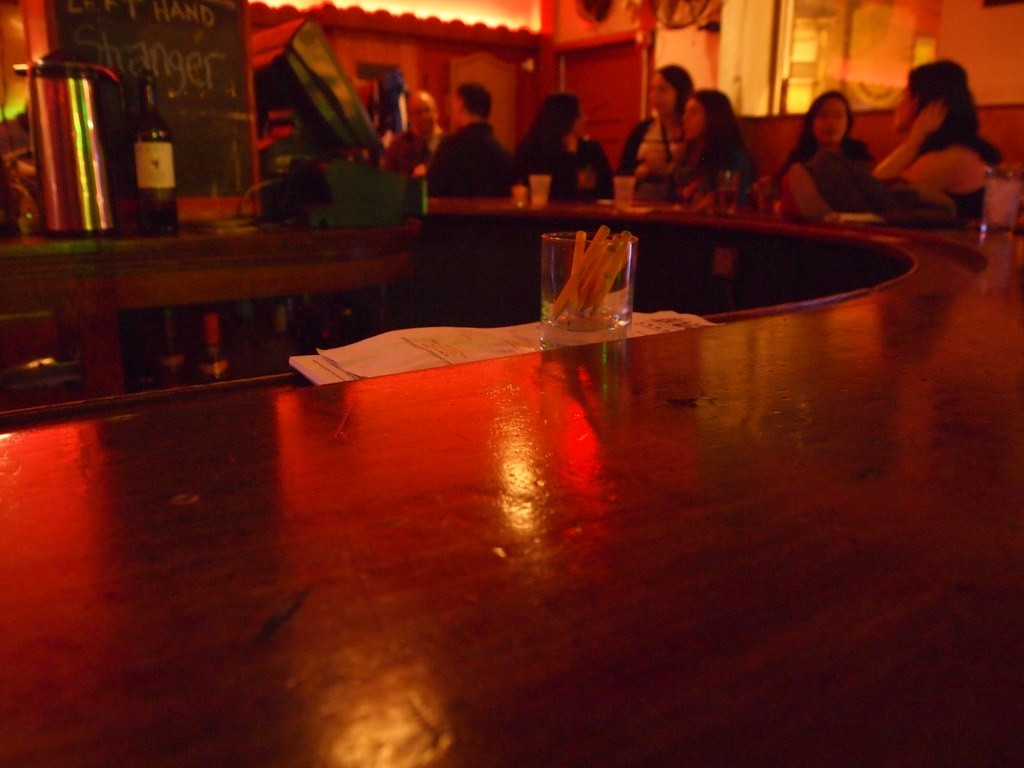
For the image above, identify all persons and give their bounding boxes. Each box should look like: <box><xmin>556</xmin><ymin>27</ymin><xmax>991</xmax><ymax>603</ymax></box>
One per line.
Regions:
<box><xmin>513</xmin><ymin>91</ymin><xmax>614</xmax><ymax>200</ymax></box>
<box><xmin>381</xmin><ymin>90</ymin><xmax>445</xmax><ymax>180</ymax></box>
<box><xmin>870</xmin><ymin>59</ymin><xmax>1003</xmax><ymax>221</ymax></box>
<box><xmin>427</xmin><ymin>81</ymin><xmax>513</xmax><ymax>197</ymax></box>
<box><xmin>752</xmin><ymin>90</ymin><xmax>870</xmax><ymax>211</ymax></box>
<box><xmin>619</xmin><ymin>66</ymin><xmax>695</xmax><ymax>204</ymax></box>
<box><xmin>668</xmin><ymin>89</ymin><xmax>751</xmax><ymax>204</ymax></box>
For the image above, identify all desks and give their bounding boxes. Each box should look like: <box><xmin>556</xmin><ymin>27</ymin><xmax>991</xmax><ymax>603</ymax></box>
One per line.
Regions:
<box><xmin>1</xmin><ymin>190</ymin><xmax>1024</xmax><ymax>768</ymax></box>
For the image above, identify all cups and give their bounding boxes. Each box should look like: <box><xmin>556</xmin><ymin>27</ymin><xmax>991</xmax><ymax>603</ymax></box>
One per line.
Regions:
<box><xmin>528</xmin><ymin>174</ymin><xmax>551</xmax><ymax>207</ymax></box>
<box><xmin>979</xmin><ymin>167</ymin><xmax>1020</xmax><ymax>248</ymax></box>
<box><xmin>717</xmin><ymin>170</ymin><xmax>739</xmax><ymax>216</ymax></box>
<box><xmin>614</xmin><ymin>176</ymin><xmax>636</xmax><ymax>210</ymax></box>
<box><xmin>540</xmin><ymin>231</ymin><xmax>639</xmax><ymax>353</ymax></box>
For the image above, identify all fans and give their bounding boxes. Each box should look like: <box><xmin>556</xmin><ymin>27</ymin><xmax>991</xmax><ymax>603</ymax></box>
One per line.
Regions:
<box><xmin>646</xmin><ymin>0</ymin><xmax>720</xmax><ymax>34</ymax></box>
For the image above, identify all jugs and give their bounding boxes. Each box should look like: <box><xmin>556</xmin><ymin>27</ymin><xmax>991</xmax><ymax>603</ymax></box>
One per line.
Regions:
<box><xmin>13</xmin><ymin>51</ymin><xmax>124</xmax><ymax>234</ymax></box>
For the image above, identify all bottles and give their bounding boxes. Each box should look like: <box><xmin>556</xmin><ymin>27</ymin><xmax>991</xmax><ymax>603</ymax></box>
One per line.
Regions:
<box><xmin>153</xmin><ymin>296</ymin><xmax>360</xmax><ymax>388</ymax></box>
<box><xmin>133</xmin><ymin>69</ymin><xmax>178</xmax><ymax>206</ymax></box>
